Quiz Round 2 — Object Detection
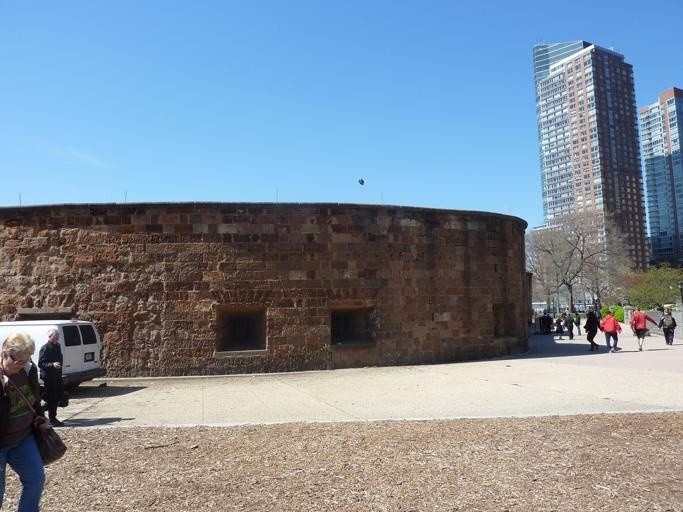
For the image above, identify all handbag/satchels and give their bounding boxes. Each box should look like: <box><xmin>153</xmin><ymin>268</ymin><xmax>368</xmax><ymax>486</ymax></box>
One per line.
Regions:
<box><xmin>32</xmin><ymin>415</ymin><xmax>68</xmax><ymax>466</ymax></box>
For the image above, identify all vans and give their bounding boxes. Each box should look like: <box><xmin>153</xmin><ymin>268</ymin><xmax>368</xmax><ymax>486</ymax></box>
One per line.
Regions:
<box><xmin>0</xmin><ymin>318</ymin><xmax>108</xmax><ymax>392</ymax></box>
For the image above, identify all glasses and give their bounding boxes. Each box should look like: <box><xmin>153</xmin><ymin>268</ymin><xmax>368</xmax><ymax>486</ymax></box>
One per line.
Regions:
<box><xmin>9</xmin><ymin>355</ymin><xmax>28</xmax><ymax>366</ymax></box>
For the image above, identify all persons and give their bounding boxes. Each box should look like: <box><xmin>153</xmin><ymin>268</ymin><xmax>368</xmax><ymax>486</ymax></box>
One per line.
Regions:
<box><xmin>1</xmin><ymin>332</ymin><xmax>49</xmax><ymax>512</ymax></box>
<box><xmin>535</xmin><ymin>304</ymin><xmax>677</xmax><ymax>352</ymax></box>
<box><xmin>39</xmin><ymin>328</ymin><xmax>64</xmax><ymax>426</ymax></box>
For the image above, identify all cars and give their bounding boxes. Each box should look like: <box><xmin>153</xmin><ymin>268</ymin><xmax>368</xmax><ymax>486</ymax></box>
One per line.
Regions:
<box><xmin>536</xmin><ymin>303</ymin><xmax>598</xmax><ymax>315</ymax></box>
